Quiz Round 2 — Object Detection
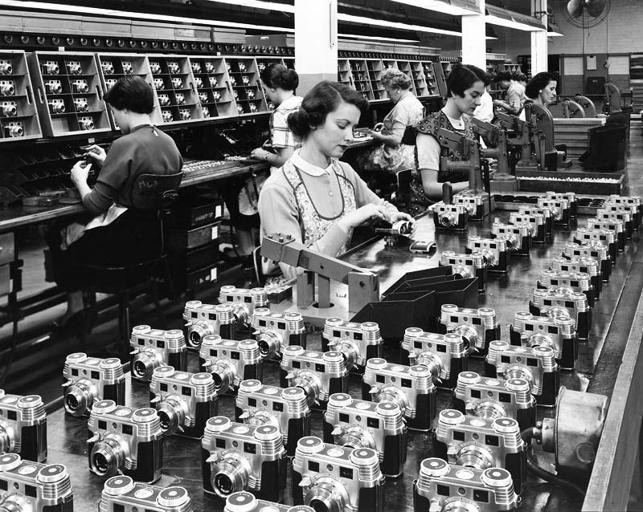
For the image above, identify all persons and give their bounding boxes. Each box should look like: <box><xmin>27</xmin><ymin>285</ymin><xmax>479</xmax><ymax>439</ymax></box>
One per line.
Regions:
<box><xmin>219</xmin><ymin>64</ymin><xmax>304</xmax><ymax>262</ymax></box>
<box><xmin>45</xmin><ymin>75</ymin><xmax>184</xmax><ymax>335</ymax></box>
<box><xmin>352</xmin><ymin>67</ymin><xmax>426</xmax><ymax>175</ymax></box>
<box><xmin>258</xmin><ymin>80</ymin><xmax>416</xmax><ymax>287</ymax></box>
<box><xmin>408</xmin><ymin>63</ymin><xmax>557</xmax><ymax>220</ymax></box>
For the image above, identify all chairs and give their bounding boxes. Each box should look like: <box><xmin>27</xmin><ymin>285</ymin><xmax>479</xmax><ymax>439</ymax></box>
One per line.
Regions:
<box><xmin>82</xmin><ymin>169</ymin><xmax>185</xmax><ymax>360</ymax></box>
<box><xmin>251</xmin><ymin>244</ymin><xmax>264</xmax><ymax>286</ymax></box>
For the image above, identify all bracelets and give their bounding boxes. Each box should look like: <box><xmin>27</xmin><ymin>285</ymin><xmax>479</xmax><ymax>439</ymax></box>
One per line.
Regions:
<box><xmin>265</xmin><ymin>152</ymin><xmax>270</xmax><ymax>160</ymax></box>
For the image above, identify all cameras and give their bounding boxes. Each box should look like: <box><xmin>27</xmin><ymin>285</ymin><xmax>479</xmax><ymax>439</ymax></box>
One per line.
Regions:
<box><xmin>321</xmin><ymin>317</ymin><xmax>385</xmax><ymax>377</ymax></box>
<box><xmin>322</xmin><ymin>392</ymin><xmax>408</xmax><ymax>478</ymax></box>
<box><xmin>199</xmin><ymin>334</ymin><xmax>263</xmax><ymax>396</ymax></box>
<box><xmin>0</xmin><ymin>389</ymin><xmax>47</xmax><ymax>462</ymax></box>
<box><xmin>182</xmin><ymin>300</ymin><xmax>236</xmax><ymax>351</ymax></box>
<box><xmin>293</xmin><ymin>436</ymin><xmax>386</xmax><ymax>512</ymax></box>
<box><xmin>61</xmin><ymin>352</ymin><xmax>125</xmax><ymax>418</ymax></box>
<box><xmin>510</xmin><ymin>311</ymin><xmax>579</xmax><ymax>370</ymax></box>
<box><xmin>338</xmin><ymin>63</ymin><xmax>436</xmax><ymax>100</ymax></box>
<box><xmin>217</xmin><ymin>286</ymin><xmax>271</xmax><ymax>331</ymax></box>
<box><xmin>529</xmin><ymin>288</ymin><xmax>591</xmax><ymax>340</ymax></box>
<box><xmin>97</xmin><ymin>475</ymin><xmax>194</xmax><ymax>512</ymax></box>
<box><xmin>40</xmin><ymin>61</ymin><xmax>276</xmax><ymax>130</ymax></box>
<box><xmin>484</xmin><ymin>340</ymin><xmax>560</xmax><ymax>407</ymax></box>
<box><xmin>87</xmin><ymin>399</ymin><xmax>163</xmax><ymax>486</ymax></box>
<box><xmin>129</xmin><ymin>325</ymin><xmax>188</xmax><ymax>384</ymax></box>
<box><xmin>0</xmin><ymin>58</ymin><xmax>24</xmax><ymax>138</ymax></box>
<box><xmin>0</xmin><ymin>452</ymin><xmax>74</xmax><ymax>512</ymax></box>
<box><xmin>412</xmin><ymin>458</ymin><xmax>523</xmax><ymax>512</ymax></box>
<box><xmin>279</xmin><ymin>345</ymin><xmax>348</xmax><ymax>411</ymax></box>
<box><xmin>149</xmin><ymin>366</ymin><xmax>218</xmax><ymax>440</ymax></box>
<box><xmin>201</xmin><ymin>415</ymin><xmax>287</xmax><ymax>500</ymax></box>
<box><xmin>438</xmin><ymin>304</ymin><xmax>501</xmax><ymax>359</ymax></box>
<box><xmin>251</xmin><ymin>308</ymin><xmax>307</xmax><ymax>361</ymax></box>
<box><xmin>362</xmin><ymin>357</ymin><xmax>438</xmax><ymax>432</ymax></box>
<box><xmin>453</xmin><ymin>371</ymin><xmax>537</xmax><ymax>445</ymax></box>
<box><xmin>431</xmin><ymin>408</ymin><xmax>524</xmax><ymax>494</ymax></box>
<box><xmin>433</xmin><ymin>189</ymin><xmax>642</xmax><ymax>294</ymax></box>
<box><xmin>224</xmin><ymin>491</ymin><xmax>316</xmax><ymax>512</ymax></box>
<box><xmin>234</xmin><ymin>379</ymin><xmax>311</xmax><ymax>459</ymax></box>
<box><xmin>401</xmin><ymin>327</ymin><xmax>465</xmax><ymax>391</ymax></box>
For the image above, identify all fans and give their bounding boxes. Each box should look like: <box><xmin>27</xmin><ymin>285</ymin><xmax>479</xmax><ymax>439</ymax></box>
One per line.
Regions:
<box><xmin>560</xmin><ymin>0</ymin><xmax>610</xmax><ymax>28</ymax></box>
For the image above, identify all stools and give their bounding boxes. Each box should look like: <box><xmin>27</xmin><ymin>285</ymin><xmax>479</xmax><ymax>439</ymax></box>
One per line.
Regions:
<box><xmin>368</xmin><ymin>168</ymin><xmax>423</xmax><ymax>217</ymax></box>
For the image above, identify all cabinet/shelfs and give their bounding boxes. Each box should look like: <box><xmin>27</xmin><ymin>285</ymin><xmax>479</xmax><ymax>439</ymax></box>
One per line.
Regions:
<box><xmin>1</xmin><ymin>48</ymin><xmax>296</xmax><ymax>147</ymax></box>
<box><xmin>338</xmin><ymin>56</ymin><xmax>524</xmax><ymax>107</ymax></box>
